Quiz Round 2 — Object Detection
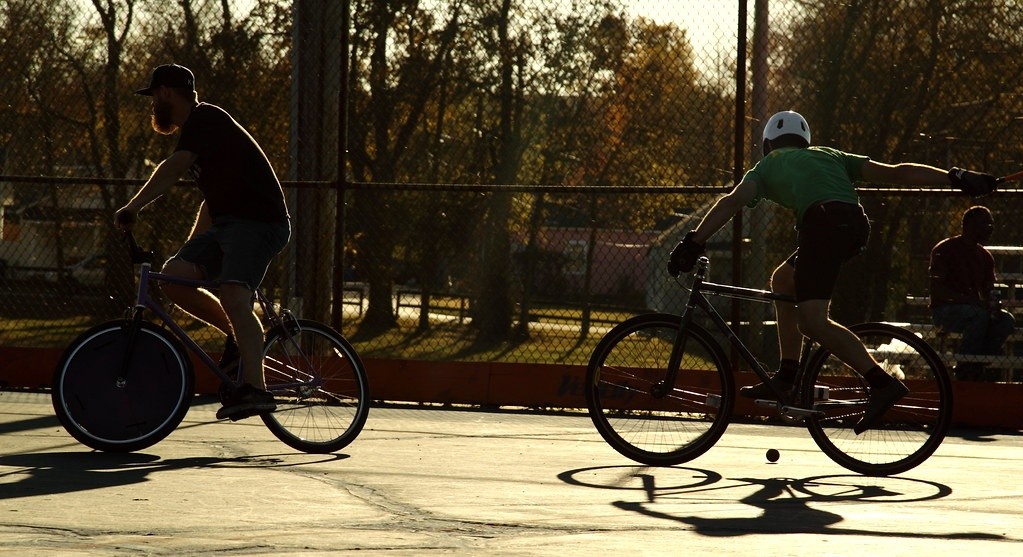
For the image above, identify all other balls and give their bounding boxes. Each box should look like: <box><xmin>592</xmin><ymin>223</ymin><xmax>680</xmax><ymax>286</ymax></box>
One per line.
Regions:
<box><xmin>767</xmin><ymin>450</ymin><xmax>779</xmax><ymax>462</ymax></box>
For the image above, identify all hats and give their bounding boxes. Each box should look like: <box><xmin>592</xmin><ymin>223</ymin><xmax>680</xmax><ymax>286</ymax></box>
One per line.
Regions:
<box><xmin>134</xmin><ymin>64</ymin><xmax>195</xmax><ymax>95</ymax></box>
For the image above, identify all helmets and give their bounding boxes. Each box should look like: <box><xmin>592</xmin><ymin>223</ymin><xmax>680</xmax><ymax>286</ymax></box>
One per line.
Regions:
<box><xmin>764</xmin><ymin>111</ymin><xmax>811</xmax><ymax>157</ymax></box>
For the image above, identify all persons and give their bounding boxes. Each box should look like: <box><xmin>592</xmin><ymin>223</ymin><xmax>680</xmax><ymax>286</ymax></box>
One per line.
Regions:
<box><xmin>930</xmin><ymin>206</ymin><xmax>1015</xmax><ymax>383</ymax></box>
<box><xmin>113</xmin><ymin>64</ymin><xmax>291</xmax><ymax>419</ymax></box>
<box><xmin>666</xmin><ymin>110</ymin><xmax>998</xmax><ymax>434</ymax></box>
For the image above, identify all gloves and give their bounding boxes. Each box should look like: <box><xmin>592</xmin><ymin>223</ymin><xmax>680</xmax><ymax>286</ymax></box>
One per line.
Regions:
<box><xmin>668</xmin><ymin>231</ymin><xmax>706</xmax><ymax>277</ymax></box>
<box><xmin>948</xmin><ymin>167</ymin><xmax>998</xmax><ymax>199</ymax></box>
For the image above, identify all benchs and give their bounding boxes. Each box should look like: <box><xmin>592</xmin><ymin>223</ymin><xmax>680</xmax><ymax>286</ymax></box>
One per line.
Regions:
<box><xmin>868</xmin><ymin>245</ymin><xmax>1023</xmax><ymax>384</ymax></box>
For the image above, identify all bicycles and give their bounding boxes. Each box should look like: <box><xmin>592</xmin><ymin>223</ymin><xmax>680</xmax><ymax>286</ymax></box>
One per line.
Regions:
<box><xmin>49</xmin><ymin>210</ymin><xmax>370</xmax><ymax>453</ymax></box>
<box><xmin>585</xmin><ymin>256</ymin><xmax>954</xmax><ymax>476</ymax></box>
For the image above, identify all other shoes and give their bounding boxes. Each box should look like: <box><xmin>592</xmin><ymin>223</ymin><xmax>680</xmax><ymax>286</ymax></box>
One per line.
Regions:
<box><xmin>215</xmin><ymin>384</ymin><xmax>275</xmax><ymax>419</ymax></box>
<box><xmin>853</xmin><ymin>376</ymin><xmax>909</xmax><ymax>435</ymax></box>
<box><xmin>739</xmin><ymin>370</ymin><xmax>799</xmax><ymax>402</ymax></box>
<box><xmin>220</xmin><ymin>332</ymin><xmax>239</xmax><ymax>367</ymax></box>
